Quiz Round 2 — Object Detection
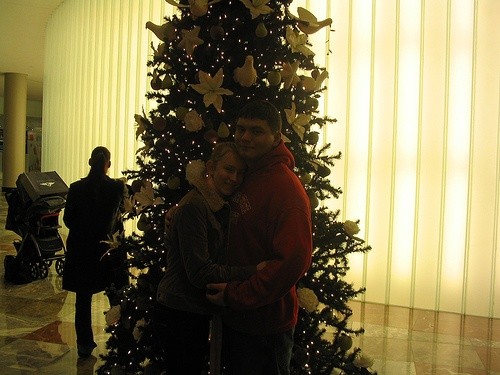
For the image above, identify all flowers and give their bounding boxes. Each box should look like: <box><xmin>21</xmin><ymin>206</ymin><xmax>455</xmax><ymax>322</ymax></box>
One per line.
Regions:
<box><xmin>98</xmin><ymin>0</ymin><xmax>375</xmax><ymax>375</ymax></box>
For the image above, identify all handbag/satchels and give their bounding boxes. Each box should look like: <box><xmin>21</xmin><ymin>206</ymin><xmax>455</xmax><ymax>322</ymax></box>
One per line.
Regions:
<box><xmin>20</xmin><ymin>171</ymin><xmax>69</xmax><ymax>203</ymax></box>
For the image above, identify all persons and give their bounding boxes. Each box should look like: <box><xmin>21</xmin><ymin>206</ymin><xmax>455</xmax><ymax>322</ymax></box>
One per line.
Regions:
<box><xmin>155</xmin><ymin>141</ymin><xmax>256</xmax><ymax>375</ymax></box>
<box><xmin>165</xmin><ymin>100</ymin><xmax>312</xmax><ymax>375</ymax></box>
<box><xmin>62</xmin><ymin>147</ymin><xmax>136</xmax><ymax>357</ymax></box>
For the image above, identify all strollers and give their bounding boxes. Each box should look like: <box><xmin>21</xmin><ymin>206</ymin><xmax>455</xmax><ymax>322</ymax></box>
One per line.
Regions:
<box><xmin>4</xmin><ymin>189</ymin><xmax>66</xmax><ymax>281</ymax></box>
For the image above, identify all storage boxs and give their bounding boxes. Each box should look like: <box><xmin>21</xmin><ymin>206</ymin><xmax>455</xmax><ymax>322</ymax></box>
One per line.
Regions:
<box><xmin>16</xmin><ymin>171</ymin><xmax>69</xmax><ymax>203</ymax></box>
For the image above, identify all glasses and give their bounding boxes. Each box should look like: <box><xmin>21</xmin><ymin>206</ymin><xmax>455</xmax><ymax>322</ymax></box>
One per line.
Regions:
<box><xmin>237</xmin><ymin>128</ymin><xmax>272</xmax><ymax>136</ymax></box>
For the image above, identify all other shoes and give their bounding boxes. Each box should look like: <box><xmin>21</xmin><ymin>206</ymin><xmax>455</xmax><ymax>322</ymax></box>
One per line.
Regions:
<box><xmin>78</xmin><ymin>342</ymin><xmax>96</xmax><ymax>357</ymax></box>
